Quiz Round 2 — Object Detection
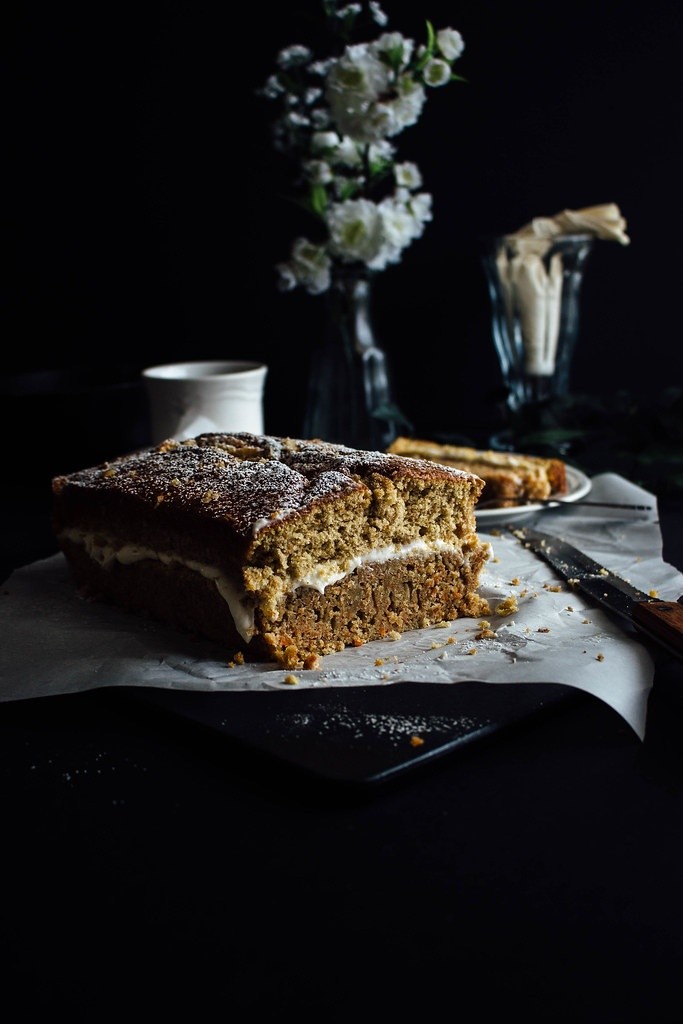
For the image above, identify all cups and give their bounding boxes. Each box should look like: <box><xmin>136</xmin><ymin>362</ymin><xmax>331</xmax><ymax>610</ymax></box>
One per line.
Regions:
<box><xmin>142</xmin><ymin>360</ymin><xmax>268</xmax><ymax>445</ymax></box>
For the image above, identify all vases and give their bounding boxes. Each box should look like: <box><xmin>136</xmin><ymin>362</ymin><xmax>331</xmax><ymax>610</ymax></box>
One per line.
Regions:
<box><xmin>302</xmin><ymin>276</ymin><xmax>417</xmax><ymax>448</ymax></box>
<box><xmin>483</xmin><ymin>234</ymin><xmax>592</xmax><ymax>461</ymax></box>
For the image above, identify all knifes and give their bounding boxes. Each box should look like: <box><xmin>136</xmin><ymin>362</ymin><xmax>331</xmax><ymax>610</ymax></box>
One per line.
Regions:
<box><xmin>505</xmin><ymin>523</ymin><xmax>683</xmax><ymax>664</ymax></box>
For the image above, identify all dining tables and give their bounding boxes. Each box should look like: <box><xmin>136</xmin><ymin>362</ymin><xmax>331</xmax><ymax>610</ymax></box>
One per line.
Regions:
<box><xmin>139</xmin><ymin>682</ymin><xmax>559</xmax><ymax>820</ymax></box>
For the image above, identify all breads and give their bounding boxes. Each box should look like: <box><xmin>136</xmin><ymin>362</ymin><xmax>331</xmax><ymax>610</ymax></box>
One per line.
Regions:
<box><xmin>387</xmin><ymin>436</ymin><xmax>566</xmax><ymax>511</ymax></box>
<box><xmin>53</xmin><ymin>431</ymin><xmax>491</xmax><ymax>668</ymax></box>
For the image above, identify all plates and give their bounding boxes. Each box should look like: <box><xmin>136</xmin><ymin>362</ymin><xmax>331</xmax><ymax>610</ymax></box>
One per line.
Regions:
<box><xmin>473</xmin><ymin>464</ymin><xmax>593</xmax><ymax>526</ymax></box>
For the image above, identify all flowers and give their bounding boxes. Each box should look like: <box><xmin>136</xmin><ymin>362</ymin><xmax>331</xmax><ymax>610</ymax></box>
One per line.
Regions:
<box><xmin>265</xmin><ymin>3</ymin><xmax>466</xmax><ymax>294</ymax></box>
